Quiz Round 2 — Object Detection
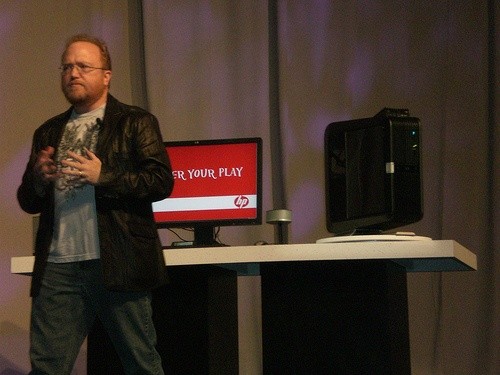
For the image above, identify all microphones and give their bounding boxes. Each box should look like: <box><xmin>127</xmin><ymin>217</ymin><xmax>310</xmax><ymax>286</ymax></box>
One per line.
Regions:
<box><xmin>97</xmin><ymin>118</ymin><xmax>103</xmax><ymax>127</ymax></box>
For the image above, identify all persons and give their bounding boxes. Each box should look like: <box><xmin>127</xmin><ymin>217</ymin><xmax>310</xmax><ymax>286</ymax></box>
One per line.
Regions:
<box><xmin>16</xmin><ymin>34</ymin><xmax>175</xmax><ymax>375</ymax></box>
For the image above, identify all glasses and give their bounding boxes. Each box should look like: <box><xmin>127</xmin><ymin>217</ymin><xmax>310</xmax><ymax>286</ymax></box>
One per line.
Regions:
<box><xmin>58</xmin><ymin>63</ymin><xmax>109</xmax><ymax>73</ymax></box>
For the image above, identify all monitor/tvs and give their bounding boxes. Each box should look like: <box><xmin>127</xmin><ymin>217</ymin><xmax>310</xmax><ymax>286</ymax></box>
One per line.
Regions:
<box><xmin>152</xmin><ymin>137</ymin><xmax>262</xmax><ymax>248</ymax></box>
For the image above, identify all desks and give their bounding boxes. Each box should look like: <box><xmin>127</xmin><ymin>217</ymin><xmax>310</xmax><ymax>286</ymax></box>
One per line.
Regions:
<box><xmin>10</xmin><ymin>240</ymin><xmax>479</xmax><ymax>375</ymax></box>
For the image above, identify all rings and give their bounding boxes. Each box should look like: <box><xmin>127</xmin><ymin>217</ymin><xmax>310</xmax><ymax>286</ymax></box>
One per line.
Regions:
<box><xmin>78</xmin><ymin>171</ymin><xmax>84</xmax><ymax>175</ymax></box>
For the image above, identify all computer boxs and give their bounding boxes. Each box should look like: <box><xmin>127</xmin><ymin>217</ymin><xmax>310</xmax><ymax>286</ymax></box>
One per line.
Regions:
<box><xmin>323</xmin><ymin>107</ymin><xmax>423</xmax><ymax>236</ymax></box>
